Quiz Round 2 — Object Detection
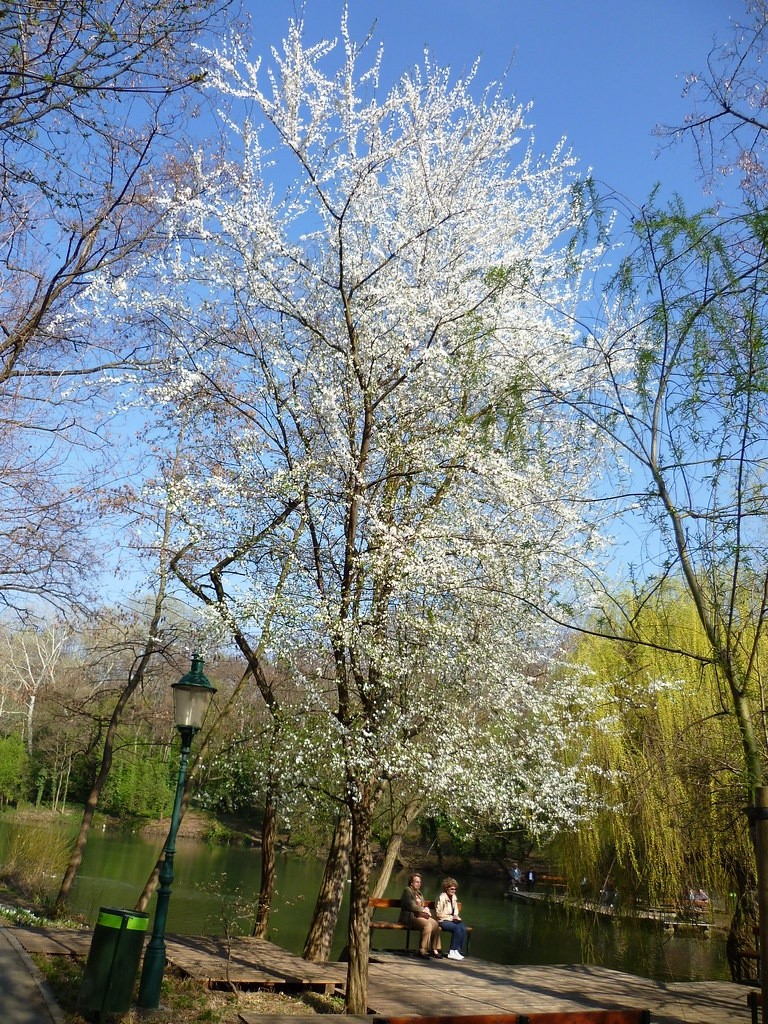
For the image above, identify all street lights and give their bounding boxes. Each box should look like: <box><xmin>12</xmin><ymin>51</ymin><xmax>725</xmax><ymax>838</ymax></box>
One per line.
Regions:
<box><xmin>133</xmin><ymin>651</ymin><xmax>218</xmax><ymax>1008</ymax></box>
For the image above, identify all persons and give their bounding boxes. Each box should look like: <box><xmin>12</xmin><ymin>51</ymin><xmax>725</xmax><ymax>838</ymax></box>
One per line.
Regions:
<box><xmin>510</xmin><ymin>863</ymin><xmax>522</xmax><ymax>892</ymax></box>
<box><xmin>604</xmin><ymin>877</ymin><xmax>616</xmax><ymax>907</ymax></box>
<box><xmin>398</xmin><ymin>873</ymin><xmax>443</xmax><ymax>959</ymax></box>
<box><xmin>686</xmin><ymin>889</ymin><xmax>709</xmax><ymax>901</ymax></box>
<box><xmin>434</xmin><ymin>877</ymin><xmax>467</xmax><ymax>960</ymax></box>
<box><xmin>525</xmin><ymin>868</ymin><xmax>538</xmax><ymax>892</ymax></box>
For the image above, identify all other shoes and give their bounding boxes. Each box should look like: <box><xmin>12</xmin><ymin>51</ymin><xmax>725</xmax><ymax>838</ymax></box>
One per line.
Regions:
<box><xmin>419</xmin><ymin>950</ymin><xmax>430</xmax><ymax>960</ymax></box>
<box><xmin>432</xmin><ymin>950</ymin><xmax>443</xmax><ymax>959</ymax></box>
<box><xmin>448</xmin><ymin>950</ymin><xmax>464</xmax><ymax>960</ymax></box>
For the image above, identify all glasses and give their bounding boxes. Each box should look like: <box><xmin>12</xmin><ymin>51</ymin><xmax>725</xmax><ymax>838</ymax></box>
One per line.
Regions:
<box><xmin>447</xmin><ymin>888</ymin><xmax>457</xmax><ymax>890</ymax></box>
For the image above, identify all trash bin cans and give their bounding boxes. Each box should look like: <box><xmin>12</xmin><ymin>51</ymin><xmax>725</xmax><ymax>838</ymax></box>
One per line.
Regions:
<box><xmin>79</xmin><ymin>906</ymin><xmax>151</xmax><ymax>1024</ymax></box>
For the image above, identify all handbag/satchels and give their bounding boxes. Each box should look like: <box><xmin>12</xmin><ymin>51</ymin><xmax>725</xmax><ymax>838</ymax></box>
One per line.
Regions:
<box><xmin>415</xmin><ymin>911</ymin><xmax>429</xmax><ymax>919</ymax></box>
